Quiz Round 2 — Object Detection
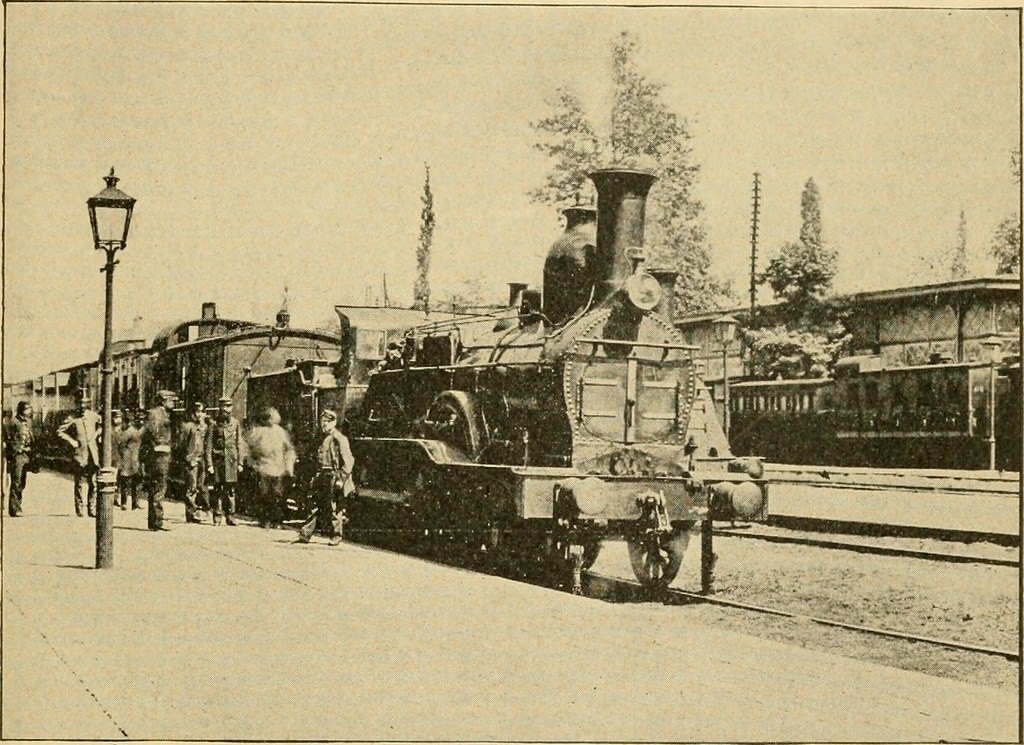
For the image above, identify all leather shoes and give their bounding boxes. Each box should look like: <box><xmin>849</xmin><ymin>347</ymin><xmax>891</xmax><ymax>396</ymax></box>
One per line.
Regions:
<box><xmin>6</xmin><ymin>494</ymin><xmax>343</xmax><ymax>546</ymax></box>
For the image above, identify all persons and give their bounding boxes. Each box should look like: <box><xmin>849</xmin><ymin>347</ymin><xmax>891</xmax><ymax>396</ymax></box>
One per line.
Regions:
<box><xmin>290</xmin><ymin>408</ymin><xmax>355</xmax><ymax>545</ymax></box>
<box><xmin>2</xmin><ymin>385</ymin><xmax>295</xmax><ymax>531</ymax></box>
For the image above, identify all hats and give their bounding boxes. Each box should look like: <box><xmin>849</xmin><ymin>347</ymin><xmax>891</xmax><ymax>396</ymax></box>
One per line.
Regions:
<box><xmin>18</xmin><ymin>389</ymin><xmax>339</xmax><ymax>422</ymax></box>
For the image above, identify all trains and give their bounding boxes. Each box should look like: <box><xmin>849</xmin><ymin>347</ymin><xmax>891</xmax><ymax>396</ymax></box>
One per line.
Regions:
<box><xmin>7</xmin><ymin>167</ymin><xmax>765</xmax><ymax>604</ymax></box>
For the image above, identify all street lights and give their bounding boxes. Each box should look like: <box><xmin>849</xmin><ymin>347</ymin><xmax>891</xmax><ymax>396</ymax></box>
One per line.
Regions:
<box><xmin>81</xmin><ymin>165</ymin><xmax>137</xmax><ymax>574</ymax></box>
